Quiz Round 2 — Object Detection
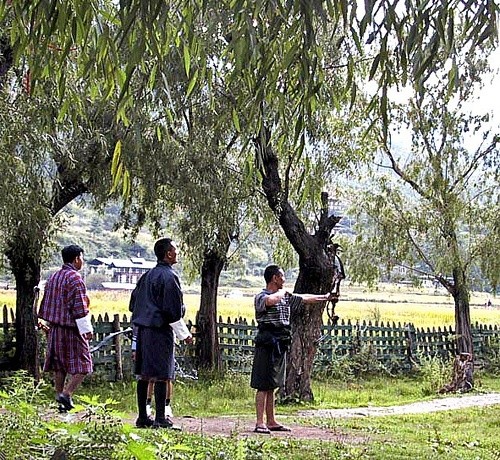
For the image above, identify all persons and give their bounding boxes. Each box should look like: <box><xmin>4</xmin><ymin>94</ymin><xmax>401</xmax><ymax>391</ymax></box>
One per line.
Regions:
<box><xmin>129</xmin><ymin>239</ymin><xmax>194</xmax><ymax>428</ymax></box>
<box><xmin>36</xmin><ymin>245</ymin><xmax>94</xmax><ymax>412</ymax></box>
<box><xmin>250</xmin><ymin>264</ymin><xmax>333</xmax><ymax>433</ymax></box>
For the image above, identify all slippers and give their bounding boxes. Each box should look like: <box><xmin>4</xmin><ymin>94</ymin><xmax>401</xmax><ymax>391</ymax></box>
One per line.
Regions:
<box><xmin>253</xmin><ymin>423</ymin><xmax>292</xmax><ymax>433</ymax></box>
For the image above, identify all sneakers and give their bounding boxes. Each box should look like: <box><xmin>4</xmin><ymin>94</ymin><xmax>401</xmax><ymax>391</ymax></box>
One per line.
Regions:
<box><xmin>145</xmin><ymin>403</ymin><xmax>152</xmax><ymax>416</ymax></box>
<box><xmin>164</xmin><ymin>405</ymin><xmax>174</xmax><ymax>418</ymax></box>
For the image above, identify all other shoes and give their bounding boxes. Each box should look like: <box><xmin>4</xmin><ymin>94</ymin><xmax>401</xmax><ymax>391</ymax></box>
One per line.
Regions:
<box><xmin>136</xmin><ymin>417</ymin><xmax>173</xmax><ymax>427</ymax></box>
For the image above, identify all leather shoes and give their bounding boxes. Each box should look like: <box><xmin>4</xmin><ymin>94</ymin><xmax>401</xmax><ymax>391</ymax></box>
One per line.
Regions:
<box><xmin>49</xmin><ymin>393</ymin><xmax>75</xmax><ymax>412</ymax></box>
